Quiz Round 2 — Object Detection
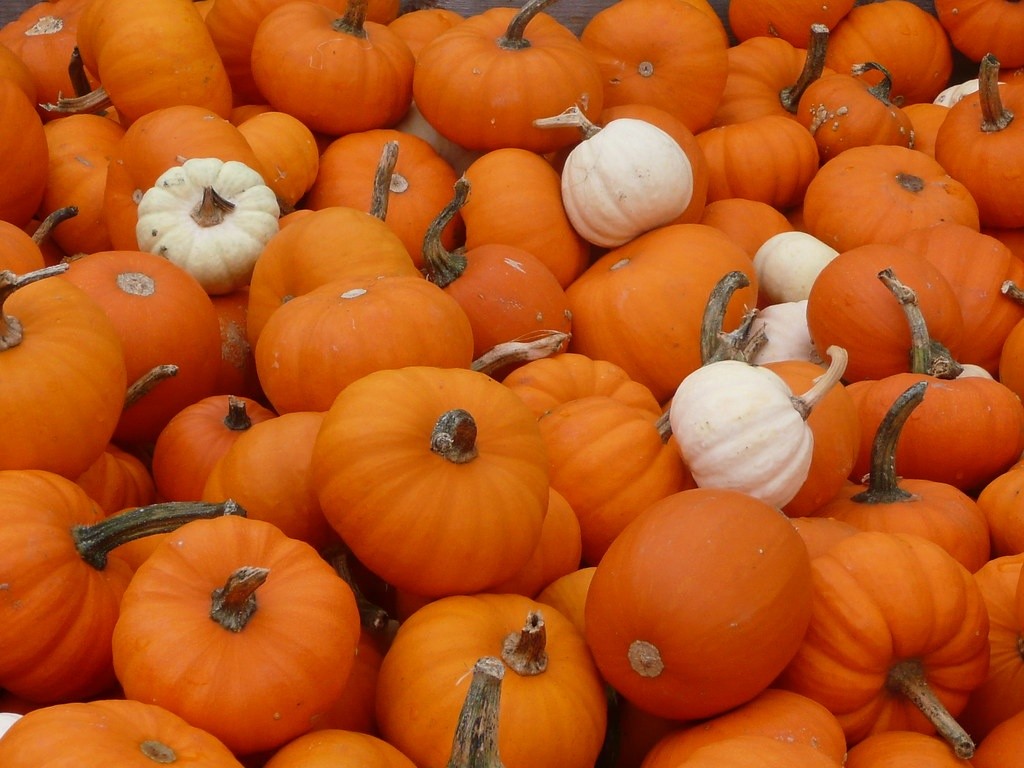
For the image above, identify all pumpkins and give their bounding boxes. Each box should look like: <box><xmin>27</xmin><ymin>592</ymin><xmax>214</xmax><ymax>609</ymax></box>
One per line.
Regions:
<box><xmin>0</xmin><ymin>0</ymin><xmax>1023</xmax><ymax>768</ymax></box>
